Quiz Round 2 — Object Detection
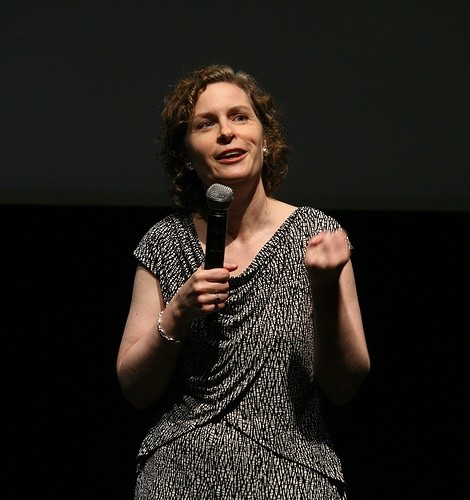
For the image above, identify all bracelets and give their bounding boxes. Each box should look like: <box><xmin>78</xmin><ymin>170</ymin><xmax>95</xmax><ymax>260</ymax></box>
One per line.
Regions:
<box><xmin>157</xmin><ymin>311</ymin><xmax>187</xmax><ymax>346</ymax></box>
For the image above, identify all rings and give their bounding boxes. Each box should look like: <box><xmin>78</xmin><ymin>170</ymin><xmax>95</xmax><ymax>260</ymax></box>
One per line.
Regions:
<box><xmin>215</xmin><ymin>294</ymin><xmax>220</xmax><ymax>302</ymax></box>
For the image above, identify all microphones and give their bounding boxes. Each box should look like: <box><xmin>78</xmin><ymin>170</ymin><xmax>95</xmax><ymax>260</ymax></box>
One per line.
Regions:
<box><xmin>204</xmin><ymin>184</ymin><xmax>234</xmax><ymax>271</ymax></box>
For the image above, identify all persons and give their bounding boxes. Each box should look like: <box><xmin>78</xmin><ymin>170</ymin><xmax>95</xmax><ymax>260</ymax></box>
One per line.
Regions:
<box><xmin>116</xmin><ymin>63</ymin><xmax>371</xmax><ymax>500</ymax></box>
